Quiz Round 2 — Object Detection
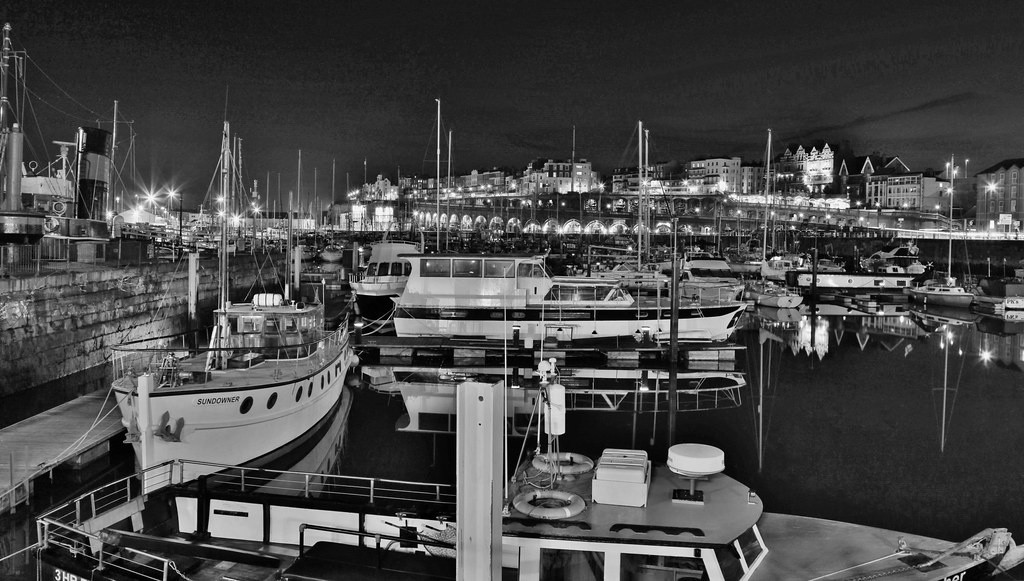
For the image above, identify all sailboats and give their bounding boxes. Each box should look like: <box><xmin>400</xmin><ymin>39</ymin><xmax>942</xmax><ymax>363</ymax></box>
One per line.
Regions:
<box><xmin>32</xmin><ymin>97</ymin><xmax>1024</xmax><ymax>581</ymax></box>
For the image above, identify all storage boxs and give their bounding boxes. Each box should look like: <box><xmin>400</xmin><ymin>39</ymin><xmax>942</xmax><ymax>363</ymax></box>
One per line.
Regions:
<box><xmin>592</xmin><ymin>448</ymin><xmax>651</xmax><ymax>507</ymax></box>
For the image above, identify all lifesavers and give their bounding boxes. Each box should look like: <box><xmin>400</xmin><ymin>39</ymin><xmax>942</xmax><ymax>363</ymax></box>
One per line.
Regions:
<box><xmin>441</xmin><ymin>370</ymin><xmax>478</xmax><ymax>382</ymax></box>
<box><xmin>513</xmin><ymin>490</ymin><xmax>587</xmax><ymax>522</ymax></box>
<box><xmin>532</xmin><ymin>452</ymin><xmax>595</xmax><ymax>475</ymax></box>
<box><xmin>43</xmin><ymin>217</ymin><xmax>60</xmax><ymax>232</ymax></box>
